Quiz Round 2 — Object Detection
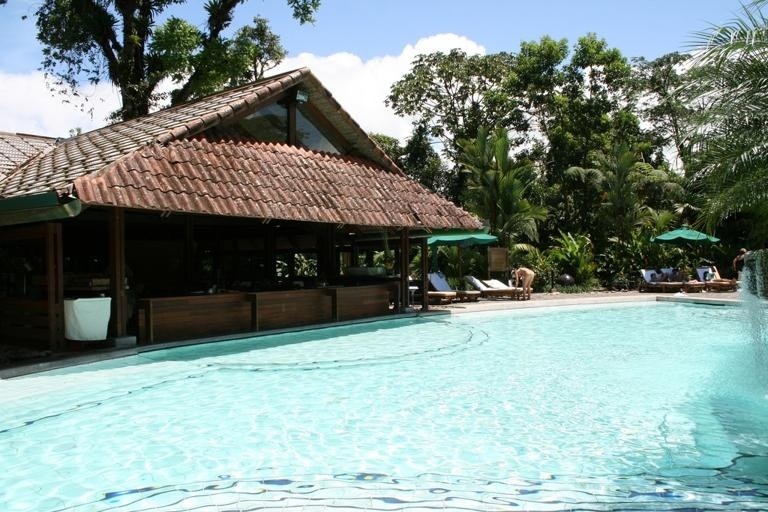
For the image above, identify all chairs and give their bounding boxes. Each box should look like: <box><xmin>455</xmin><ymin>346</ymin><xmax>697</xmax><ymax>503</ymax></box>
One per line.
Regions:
<box><xmin>639</xmin><ymin>266</ymin><xmax>739</xmax><ymax>293</ymax></box>
<box><xmin>427</xmin><ymin>271</ymin><xmax>534</xmax><ymax>304</ymax></box>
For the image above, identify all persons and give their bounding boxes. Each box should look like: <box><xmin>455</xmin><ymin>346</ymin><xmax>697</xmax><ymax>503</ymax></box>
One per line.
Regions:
<box><xmin>511</xmin><ymin>267</ymin><xmax>535</xmax><ymax>300</ymax></box>
<box><xmin>651</xmin><ymin>269</ymin><xmax>700</xmax><ymax>282</ymax></box>
<box><xmin>733</xmin><ymin>248</ymin><xmax>747</xmax><ymax>281</ymax></box>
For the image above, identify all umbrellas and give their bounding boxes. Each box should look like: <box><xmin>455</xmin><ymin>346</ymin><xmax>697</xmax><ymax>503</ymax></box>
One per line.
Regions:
<box><xmin>427</xmin><ymin>233</ymin><xmax>498</xmax><ymax>292</ymax></box>
<box><xmin>649</xmin><ymin>229</ymin><xmax>721</xmax><ymax>273</ymax></box>
